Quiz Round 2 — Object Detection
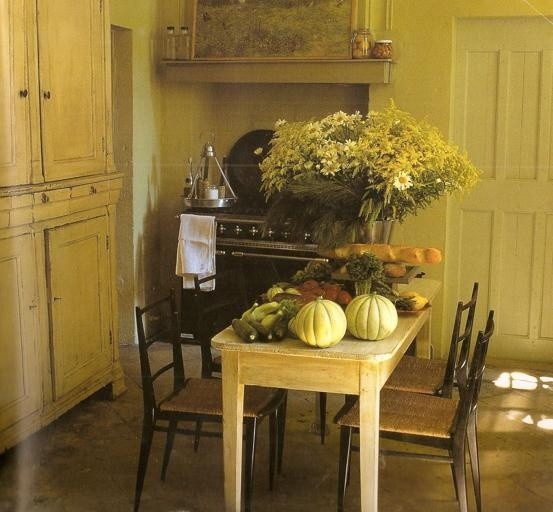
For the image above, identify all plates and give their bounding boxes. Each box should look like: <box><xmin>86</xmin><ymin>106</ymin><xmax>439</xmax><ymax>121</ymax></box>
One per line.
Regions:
<box><xmin>397</xmin><ymin>301</ymin><xmax>432</xmax><ymax>313</ymax></box>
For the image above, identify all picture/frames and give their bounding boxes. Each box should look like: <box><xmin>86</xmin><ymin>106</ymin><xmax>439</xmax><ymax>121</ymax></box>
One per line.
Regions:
<box><xmin>190</xmin><ymin>0</ymin><xmax>359</xmax><ymax>59</ymax></box>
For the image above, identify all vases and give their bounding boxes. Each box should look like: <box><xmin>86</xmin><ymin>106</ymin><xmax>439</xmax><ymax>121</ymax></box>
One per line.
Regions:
<box><xmin>360</xmin><ymin>219</ymin><xmax>393</xmax><ymax>245</ymax></box>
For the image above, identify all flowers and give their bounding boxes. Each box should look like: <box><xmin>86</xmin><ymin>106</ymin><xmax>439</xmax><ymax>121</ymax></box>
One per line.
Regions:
<box><xmin>258</xmin><ymin>106</ymin><xmax>485</xmax><ymax>252</ymax></box>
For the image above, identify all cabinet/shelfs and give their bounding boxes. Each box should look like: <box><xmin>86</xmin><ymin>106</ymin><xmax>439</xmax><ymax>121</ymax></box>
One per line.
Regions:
<box><xmin>0</xmin><ymin>0</ymin><xmax>116</xmax><ymax>192</ymax></box>
<box><xmin>0</xmin><ymin>172</ymin><xmax>128</xmax><ymax>455</ymax></box>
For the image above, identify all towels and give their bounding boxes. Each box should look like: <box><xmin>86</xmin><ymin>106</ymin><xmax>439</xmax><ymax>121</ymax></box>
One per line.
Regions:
<box><xmin>175</xmin><ymin>214</ymin><xmax>216</xmax><ymax>291</ymax></box>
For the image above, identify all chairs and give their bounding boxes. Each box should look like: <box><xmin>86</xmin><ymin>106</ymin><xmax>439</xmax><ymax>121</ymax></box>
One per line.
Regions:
<box><xmin>332</xmin><ymin>309</ymin><xmax>496</xmax><ymax>512</ymax></box>
<box><xmin>345</xmin><ymin>282</ymin><xmax>479</xmax><ymax>500</ymax></box>
<box><xmin>193</xmin><ymin>267</ymin><xmax>327</xmax><ymax>475</ymax></box>
<box><xmin>134</xmin><ymin>288</ymin><xmax>284</xmax><ymax>512</ymax></box>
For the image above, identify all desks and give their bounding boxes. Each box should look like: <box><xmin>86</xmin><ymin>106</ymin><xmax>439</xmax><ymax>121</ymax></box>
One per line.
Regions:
<box><xmin>209</xmin><ymin>278</ymin><xmax>443</xmax><ymax>512</ymax></box>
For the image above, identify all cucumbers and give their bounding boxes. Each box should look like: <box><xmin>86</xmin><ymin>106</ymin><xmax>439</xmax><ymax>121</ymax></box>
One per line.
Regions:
<box><xmin>248</xmin><ymin>319</ymin><xmax>274</xmax><ymax>342</ymax></box>
<box><xmin>231</xmin><ymin>318</ymin><xmax>259</xmax><ymax>342</ymax></box>
<box><xmin>273</xmin><ymin>320</ymin><xmax>288</xmax><ymax>340</ymax></box>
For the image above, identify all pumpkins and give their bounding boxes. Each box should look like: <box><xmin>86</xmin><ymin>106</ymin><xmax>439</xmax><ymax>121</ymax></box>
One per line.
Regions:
<box><xmin>295</xmin><ymin>296</ymin><xmax>348</xmax><ymax>349</ymax></box>
<box><xmin>344</xmin><ymin>290</ymin><xmax>399</xmax><ymax>341</ymax></box>
